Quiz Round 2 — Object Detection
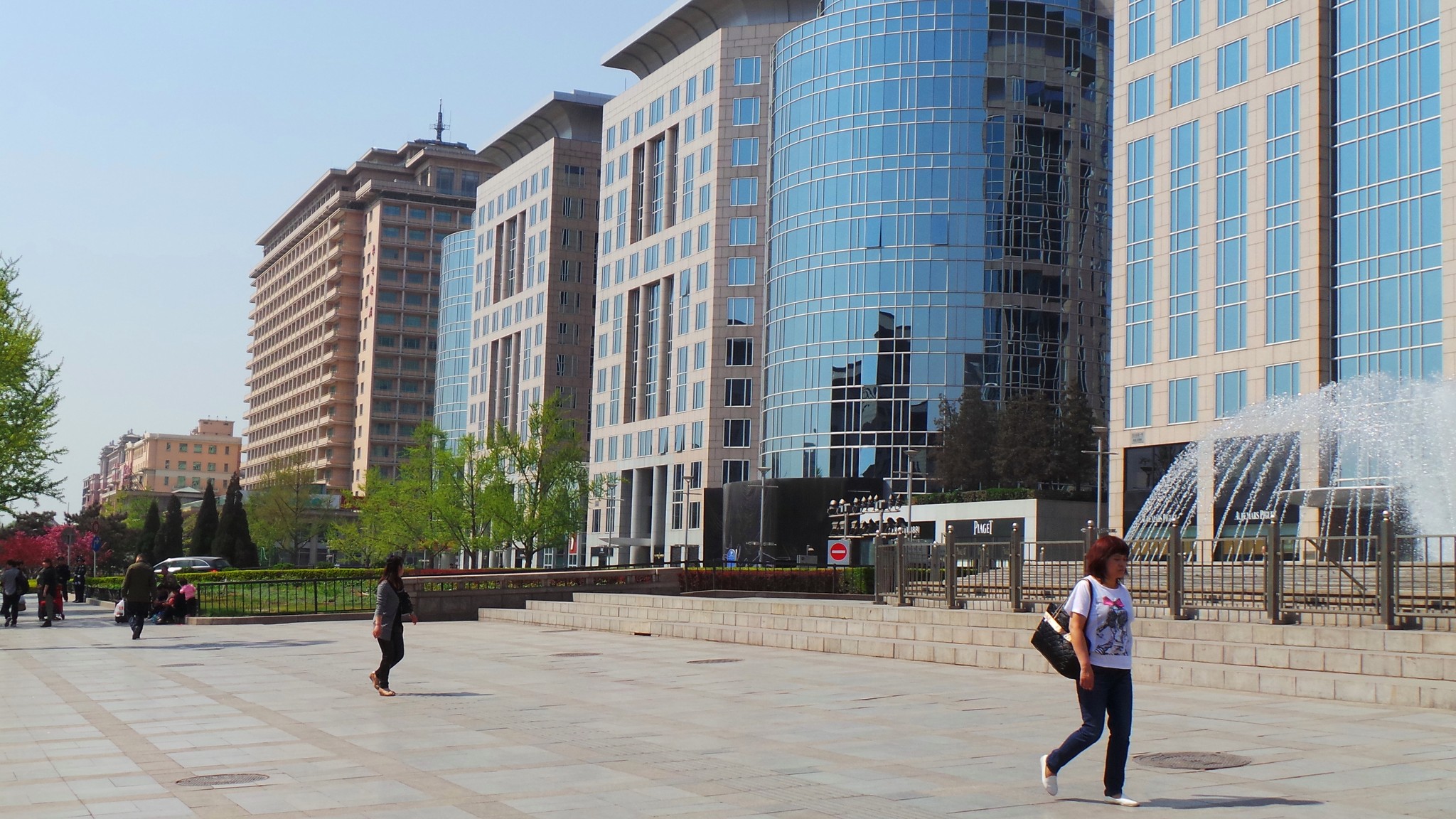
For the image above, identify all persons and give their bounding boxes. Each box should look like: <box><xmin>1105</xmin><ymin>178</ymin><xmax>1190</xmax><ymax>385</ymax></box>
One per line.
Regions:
<box><xmin>56</xmin><ymin>557</ymin><xmax>70</xmax><ymax>602</ymax></box>
<box><xmin>160</xmin><ymin>564</ymin><xmax>177</xmax><ymax>593</ymax></box>
<box><xmin>121</xmin><ymin>553</ymin><xmax>157</xmax><ymax>639</ymax></box>
<box><xmin>147</xmin><ymin>583</ymin><xmax>170</xmax><ymax>621</ymax></box>
<box><xmin>155</xmin><ymin>584</ymin><xmax>188</xmax><ymax>625</ymax></box>
<box><xmin>0</xmin><ymin>561</ymin><xmax>22</xmax><ymax>615</ymax></box>
<box><xmin>693</xmin><ymin>559</ymin><xmax>700</xmax><ymax>568</ymax></box>
<box><xmin>37</xmin><ymin>567</ymin><xmax>61</xmax><ymax>621</ymax></box>
<box><xmin>179</xmin><ymin>578</ymin><xmax>197</xmax><ymax>617</ymax></box>
<box><xmin>368</xmin><ymin>555</ymin><xmax>417</xmax><ymax>696</ymax></box>
<box><xmin>72</xmin><ymin>556</ymin><xmax>86</xmax><ymax>603</ymax></box>
<box><xmin>447</xmin><ymin>563</ymin><xmax>455</xmax><ymax>569</ymax></box>
<box><xmin>1039</xmin><ymin>535</ymin><xmax>1140</xmax><ymax>807</ymax></box>
<box><xmin>40</xmin><ymin>558</ymin><xmax>55</xmax><ymax>627</ymax></box>
<box><xmin>0</xmin><ymin>560</ymin><xmax>23</xmax><ymax>627</ymax></box>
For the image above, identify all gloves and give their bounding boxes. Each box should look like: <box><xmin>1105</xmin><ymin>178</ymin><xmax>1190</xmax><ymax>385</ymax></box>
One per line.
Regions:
<box><xmin>78</xmin><ymin>581</ymin><xmax>81</xmax><ymax>584</ymax></box>
<box><xmin>75</xmin><ymin>582</ymin><xmax>78</xmax><ymax>585</ymax></box>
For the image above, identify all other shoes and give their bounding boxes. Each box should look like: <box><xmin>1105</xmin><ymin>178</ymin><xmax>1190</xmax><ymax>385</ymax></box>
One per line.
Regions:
<box><xmin>65</xmin><ymin>600</ymin><xmax>67</xmax><ymax>602</ymax></box>
<box><xmin>1040</xmin><ymin>754</ymin><xmax>1064</xmax><ymax>796</ymax></box>
<box><xmin>133</xmin><ymin>626</ymin><xmax>139</xmax><ymax>640</ymax></box>
<box><xmin>72</xmin><ymin>600</ymin><xmax>77</xmax><ymax>602</ymax></box>
<box><xmin>155</xmin><ymin>619</ymin><xmax>169</xmax><ymax>624</ymax></box>
<box><xmin>147</xmin><ymin>617</ymin><xmax>153</xmax><ymax>620</ymax></box>
<box><xmin>5</xmin><ymin>616</ymin><xmax>11</xmax><ymax>627</ymax></box>
<box><xmin>369</xmin><ymin>671</ymin><xmax>380</xmax><ymax>690</ymax></box>
<box><xmin>75</xmin><ymin>600</ymin><xmax>83</xmax><ymax>602</ymax></box>
<box><xmin>379</xmin><ymin>685</ymin><xmax>395</xmax><ymax>696</ymax></box>
<box><xmin>52</xmin><ymin>617</ymin><xmax>60</xmax><ymax>621</ymax></box>
<box><xmin>1105</xmin><ymin>792</ymin><xmax>1140</xmax><ymax>807</ymax></box>
<box><xmin>40</xmin><ymin>622</ymin><xmax>51</xmax><ymax>627</ymax></box>
<box><xmin>11</xmin><ymin>624</ymin><xmax>16</xmax><ymax>627</ymax></box>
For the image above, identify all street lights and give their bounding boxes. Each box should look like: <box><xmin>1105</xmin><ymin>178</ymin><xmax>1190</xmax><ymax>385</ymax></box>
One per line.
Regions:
<box><xmin>1081</xmin><ymin>426</ymin><xmax>1118</xmax><ymax>541</ymax></box>
<box><xmin>673</xmin><ymin>476</ymin><xmax>702</xmax><ymax>562</ymax></box>
<box><xmin>893</xmin><ymin>449</ymin><xmax>928</xmax><ymax>542</ymax></box>
<box><xmin>748</xmin><ymin>467</ymin><xmax>778</xmax><ymax>570</ymax></box>
<box><xmin>597</xmin><ymin>483</ymin><xmax>625</xmax><ymax>565</ymax></box>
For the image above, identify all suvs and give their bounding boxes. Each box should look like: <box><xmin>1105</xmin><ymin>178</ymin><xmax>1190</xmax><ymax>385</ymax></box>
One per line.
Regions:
<box><xmin>152</xmin><ymin>556</ymin><xmax>232</xmax><ymax>573</ymax></box>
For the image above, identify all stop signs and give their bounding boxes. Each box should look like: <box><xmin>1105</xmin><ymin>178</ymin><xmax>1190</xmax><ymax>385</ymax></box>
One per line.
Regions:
<box><xmin>830</xmin><ymin>543</ymin><xmax>847</xmax><ymax>560</ymax></box>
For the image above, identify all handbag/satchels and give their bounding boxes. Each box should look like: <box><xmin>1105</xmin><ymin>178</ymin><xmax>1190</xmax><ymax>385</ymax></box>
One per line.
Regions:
<box><xmin>400</xmin><ymin>590</ymin><xmax>413</xmax><ymax>615</ymax></box>
<box><xmin>15</xmin><ymin>572</ymin><xmax>29</xmax><ymax>596</ymax></box>
<box><xmin>114</xmin><ymin>598</ymin><xmax>125</xmax><ymax>617</ymax></box>
<box><xmin>1030</xmin><ymin>578</ymin><xmax>1094</xmax><ymax>681</ymax></box>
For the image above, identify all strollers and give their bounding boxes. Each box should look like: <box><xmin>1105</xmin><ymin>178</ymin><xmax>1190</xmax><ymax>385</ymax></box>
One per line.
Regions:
<box><xmin>41</xmin><ymin>585</ymin><xmax>65</xmax><ymax>619</ymax></box>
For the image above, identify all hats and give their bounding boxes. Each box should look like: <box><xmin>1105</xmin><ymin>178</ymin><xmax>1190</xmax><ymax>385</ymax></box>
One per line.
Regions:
<box><xmin>77</xmin><ymin>556</ymin><xmax>85</xmax><ymax>562</ymax></box>
<box><xmin>57</xmin><ymin>557</ymin><xmax>64</xmax><ymax>560</ymax></box>
